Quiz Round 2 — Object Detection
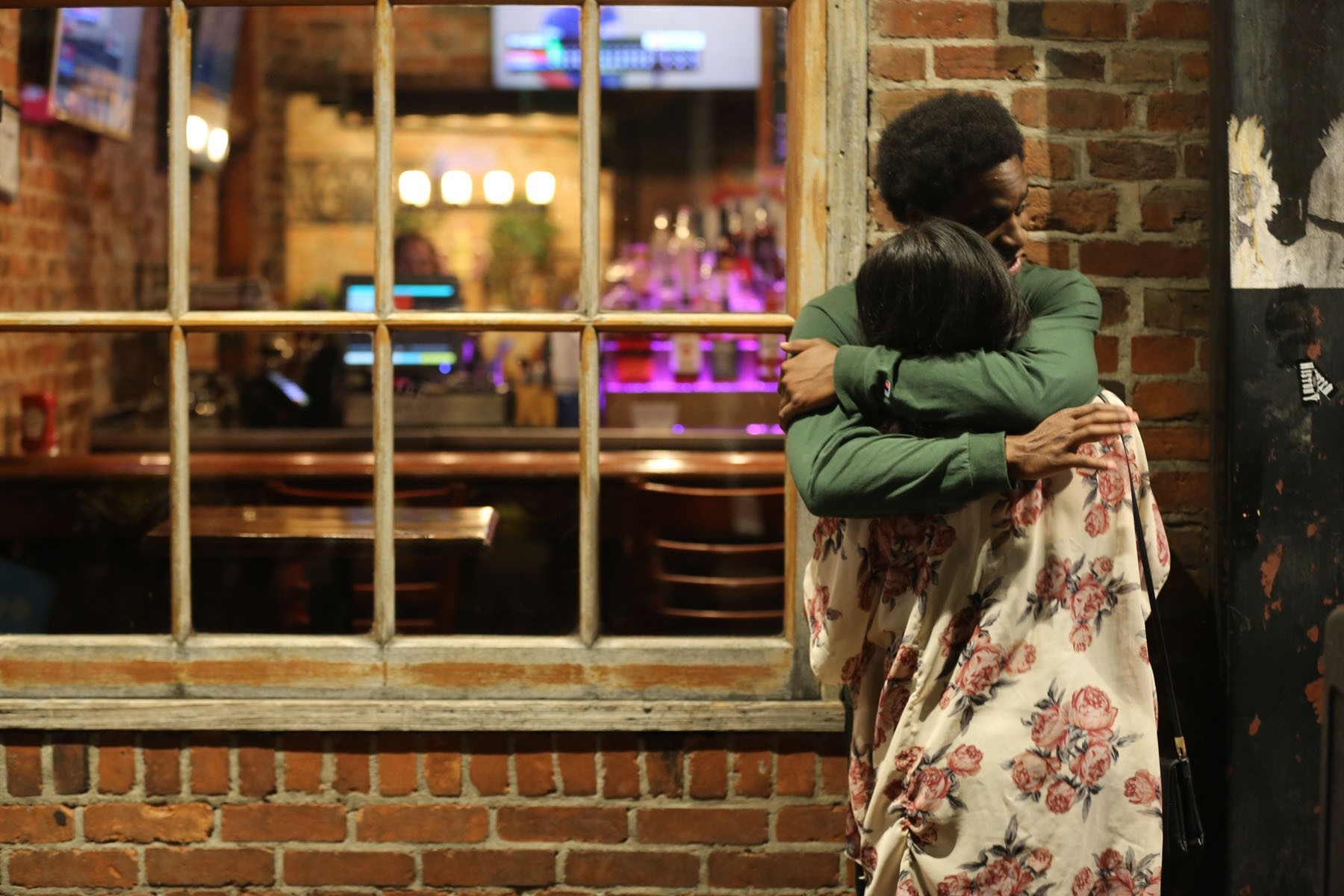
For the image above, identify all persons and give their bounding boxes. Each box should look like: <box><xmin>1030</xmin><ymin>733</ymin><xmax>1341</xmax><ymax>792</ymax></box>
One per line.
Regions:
<box><xmin>779</xmin><ymin>92</ymin><xmax>1142</xmax><ymax>896</ymax></box>
<box><xmin>392</xmin><ymin>229</ymin><xmax>439</xmax><ymax>278</ymax></box>
<box><xmin>804</xmin><ymin>217</ymin><xmax>1204</xmax><ymax>896</ymax></box>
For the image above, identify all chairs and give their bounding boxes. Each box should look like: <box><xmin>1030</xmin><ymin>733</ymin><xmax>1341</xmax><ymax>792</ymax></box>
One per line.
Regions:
<box><xmin>262</xmin><ymin>479</ymin><xmax>466</xmax><ymax>635</ymax></box>
<box><xmin>622</xmin><ymin>473</ymin><xmax>785</xmax><ymax>636</ymax></box>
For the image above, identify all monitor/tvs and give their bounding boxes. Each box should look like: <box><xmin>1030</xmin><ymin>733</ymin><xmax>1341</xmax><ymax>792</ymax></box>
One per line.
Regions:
<box><xmin>335</xmin><ymin>274</ymin><xmax>466</xmax><ymax>373</ymax></box>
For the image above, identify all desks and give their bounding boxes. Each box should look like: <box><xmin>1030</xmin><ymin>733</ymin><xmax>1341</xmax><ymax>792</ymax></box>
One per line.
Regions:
<box><xmin>0</xmin><ymin>444</ymin><xmax>786</xmax><ymax>632</ymax></box>
<box><xmin>145</xmin><ymin>501</ymin><xmax>500</xmax><ymax>635</ymax></box>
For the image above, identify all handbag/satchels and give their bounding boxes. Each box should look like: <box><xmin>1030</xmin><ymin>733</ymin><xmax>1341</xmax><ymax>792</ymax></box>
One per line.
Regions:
<box><xmin>1161</xmin><ymin>745</ymin><xmax>1205</xmax><ymax>854</ymax></box>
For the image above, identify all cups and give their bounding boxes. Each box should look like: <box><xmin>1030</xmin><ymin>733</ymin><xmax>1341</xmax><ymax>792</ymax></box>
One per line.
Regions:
<box><xmin>19</xmin><ymin>391</ymin><xmax>55</xmax><ymax>454</ymax></box>
<box><xmin>711</xmin><ymin>340</ymin><xmax>737</xmax><ymax>380</ymax></box>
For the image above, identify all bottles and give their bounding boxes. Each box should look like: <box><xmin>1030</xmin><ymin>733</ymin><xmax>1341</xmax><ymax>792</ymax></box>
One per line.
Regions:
<box><xmin>619</xmin><ymin>299</ymin><xmax>652</xmax><ymax>383</ymax></box>
<box><xmin>668</xmin><ymin>331</ymin><xmax>701</xmax><ymax>382</ymax></box>
<box><xmin>755</xmin><ymin>332</ymin><xmax>787</xmax><ymax>384</ymax></box>
